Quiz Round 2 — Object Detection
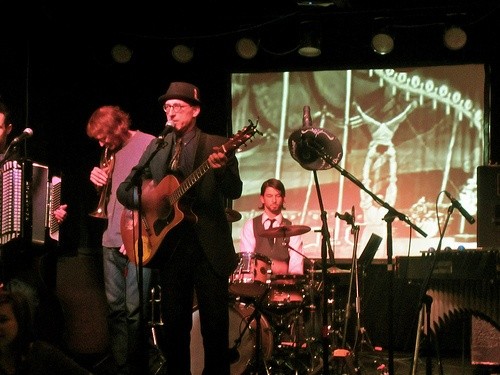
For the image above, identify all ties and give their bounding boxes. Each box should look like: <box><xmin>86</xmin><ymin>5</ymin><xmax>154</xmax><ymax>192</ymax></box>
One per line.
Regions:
<box><xmin>267</xmin><ymin>219</ymin><xmax>277</xmax><ymax>248</ymax></box>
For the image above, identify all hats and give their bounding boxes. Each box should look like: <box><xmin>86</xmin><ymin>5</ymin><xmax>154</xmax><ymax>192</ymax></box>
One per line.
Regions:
<box><xmin>159</xmin><ymin>82</ymin><xmax>203</xmax><ymax>106</ymax></box>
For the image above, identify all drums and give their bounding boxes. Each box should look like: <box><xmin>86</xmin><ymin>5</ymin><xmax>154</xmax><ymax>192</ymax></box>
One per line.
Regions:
<box><xmin>190</xmin><ymin>301</ymin><xmax>274</xmax><ymax>375</ymax></box>
<box><xmin>227</xmin><ymin>251</ymin><xmax>272</xmax><ymax>296</ymax></box>
<box><xmin>266</xmin><ymin>274</ymin><xmax>305</xmax><ymax>305</ymax></box>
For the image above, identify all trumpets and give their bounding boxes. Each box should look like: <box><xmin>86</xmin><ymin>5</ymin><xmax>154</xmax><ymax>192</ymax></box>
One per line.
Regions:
<box><xmin>89</xmin><ymin>143</ymin><xmax>116</xmax><ymax>219</ymax></box>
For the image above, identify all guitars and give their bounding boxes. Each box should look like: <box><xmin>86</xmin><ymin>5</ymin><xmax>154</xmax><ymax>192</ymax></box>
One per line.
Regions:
<box><xmin>120</xmin><ymin>119</ymin><xmax>264</xmax><ymax>267</ymax></box>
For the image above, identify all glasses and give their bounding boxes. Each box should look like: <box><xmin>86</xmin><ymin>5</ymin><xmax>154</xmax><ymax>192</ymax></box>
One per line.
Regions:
<box><xmin>162</xmin><ymin>103</ymin><xmax>187</xmax><ymax>113</ymax></box>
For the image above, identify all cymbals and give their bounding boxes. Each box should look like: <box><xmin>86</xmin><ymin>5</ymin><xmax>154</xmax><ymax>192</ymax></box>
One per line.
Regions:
<box><xmin>307</xmin><ymin>266</ymin><xmax>354</xmax><ymax>273</ymax></box>
<box><xmin>257</xmin><ymin>223</ymin><xmax>311</xmax><ymax>238</ymax></box>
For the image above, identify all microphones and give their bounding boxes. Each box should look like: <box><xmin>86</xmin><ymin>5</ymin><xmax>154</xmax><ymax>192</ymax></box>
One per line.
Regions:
<box><xmin>445</xmin><ymin>191</ymin><xmax>475</xmax><ymax>225</ymax></box>
<box><xmin>12</xmin><ymin>128</ymin><xmax>33</xmax><ymax>145</ymax></box>
<box><xmin>303</xmin><ymin>105</ymin><xmax>312</xmax><ymax>129</ymax></box>
<box><xmin>155</xmin><ymin>121</ymin><xmax>174</xmax><ymax>145</ymax></box>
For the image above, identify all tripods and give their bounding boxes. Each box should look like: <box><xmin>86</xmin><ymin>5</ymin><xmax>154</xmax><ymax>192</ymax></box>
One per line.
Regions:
<box><xmin>311</xmin><ymin>217</ymin><xmax>379</xmax><ymax>375</ymax></box>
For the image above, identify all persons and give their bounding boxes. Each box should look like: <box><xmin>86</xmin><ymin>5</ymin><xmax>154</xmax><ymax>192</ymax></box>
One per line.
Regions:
<box><xmin>0</xmin><ymin>290</ymin><xmax>93</xmax><ymax>375</ymax></box>
<box><xmin>240</xmin><ymin>179</ymin><xmax>304</xmax><ymax>276</ymax></box>
<box><xmin>351</xmin><ymin>100</ymin><xmax>429</xmax><ymax>258</ymax></box>
<box><xmin>0</xmin><ymin>104</ymin><xmax>75</xmax><ymax>357</ymax></box>
<box><xmin>116</xmin><ymin>82</ymin><xmax>243</xmax><ymax>375</ymax></box>
<box><xmin>87</xmin><ymin>106</ymin><xmax>155</xmax><ymax>369</ymax></box>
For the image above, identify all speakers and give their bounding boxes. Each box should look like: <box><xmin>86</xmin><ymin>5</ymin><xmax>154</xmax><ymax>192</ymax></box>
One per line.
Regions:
<box><xmin>477</xmin><ymin>165</ymin><xmax>500</xmax><ymax>248</ymax></box>
